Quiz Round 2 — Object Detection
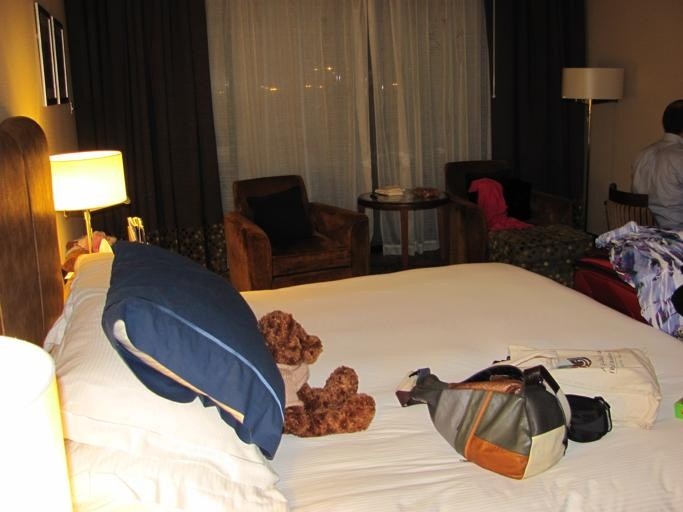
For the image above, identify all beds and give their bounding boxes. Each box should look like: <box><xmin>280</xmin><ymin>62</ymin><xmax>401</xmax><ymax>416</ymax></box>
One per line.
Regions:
<box><xmin>0</xmin><ymin>116</ymin><xmax>683</xmax><ymax>512</ymax></box>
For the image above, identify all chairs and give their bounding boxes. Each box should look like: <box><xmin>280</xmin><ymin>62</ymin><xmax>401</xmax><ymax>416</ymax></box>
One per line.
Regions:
<box><xmin>225</xmin><ymin>174</ymin><xmax>369</xmax><ymax>291</ymax></box>
<box><xmin>438</xmin><ymin>159</ymin><xmax>573</xmax><ymax>266</ymax></box>
<box><xmin>608</xmin><ymin>183</ymin><xmax>659</xmax><ymax>233</ymax></box>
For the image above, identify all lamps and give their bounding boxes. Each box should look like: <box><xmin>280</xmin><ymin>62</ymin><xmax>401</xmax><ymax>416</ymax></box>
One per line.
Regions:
<box><xmin>48</xmin><ymin>151</ymin><xmax>127</xmax><ymax>253</ymax></box>
<box><xmin>0</xmin><ymin>336</ymin><xmax>71</xmax><ymax>512</ymax></box>
<box><xmin>562</xmin><ymin>67</ymin><xmax>624</xmax><ymax>232</ymax></box>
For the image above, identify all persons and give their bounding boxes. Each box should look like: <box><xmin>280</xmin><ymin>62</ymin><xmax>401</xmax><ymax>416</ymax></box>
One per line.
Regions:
<box><xmin>631</xmin><ymin>99</ymin><xmax>683</xmax><ymax>232</ymax></box>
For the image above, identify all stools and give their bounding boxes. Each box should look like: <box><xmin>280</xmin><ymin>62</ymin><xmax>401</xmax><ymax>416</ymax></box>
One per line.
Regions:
<box><xmin>487</xmin><ymin>225</ymin><xmax>594</xmax><ymax>288</ymax></box>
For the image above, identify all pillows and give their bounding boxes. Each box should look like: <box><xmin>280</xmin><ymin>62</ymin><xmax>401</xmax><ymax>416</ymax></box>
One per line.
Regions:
<box><xmin>494</xmin><ymin>176</ymin><xmax>533</xmax><ymax>221</ymax></box>
<box><xmin>465</xmin><ymin>172</ymin><xmax>505</xmax><ymax>204</ymax></box>
<box><xmin>102</xmin><ymin>238</ymin><xmax>285</xmax><ymax>460</ymax></box>
<box><xmin>56</xmin><ymin>253</ymin><xmax>279</xmax><ymax>490</ymax></box>
<box><xmin>246</xmin><ymin>185</ymin><xmax>311</xmax><ymax>248</ymax></box>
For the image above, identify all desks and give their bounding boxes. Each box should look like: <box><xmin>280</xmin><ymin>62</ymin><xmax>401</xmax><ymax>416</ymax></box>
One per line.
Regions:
<box><xmin>357</xmin><ymin>189</ymin><xmax>450</xmax><ymax>270</ymax></box>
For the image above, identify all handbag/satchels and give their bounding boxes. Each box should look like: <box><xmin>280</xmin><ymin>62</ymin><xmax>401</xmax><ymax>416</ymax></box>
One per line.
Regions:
<box><xmin>566</xmin><ymin>395</ymin><xmax>612</xmax><ymax>443</ymax></box>
<box><xmin>491</xmin><ymin>345</ymin><xmax>661</xmax><ymax>428</ymax></box>
<box><xmin>397</xmin><ymin>364</ymin><xmax>611</xmax><ymax>479</ymax></box>
